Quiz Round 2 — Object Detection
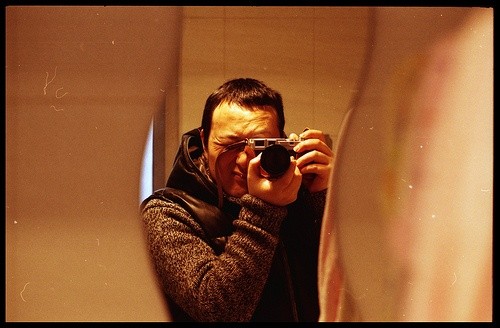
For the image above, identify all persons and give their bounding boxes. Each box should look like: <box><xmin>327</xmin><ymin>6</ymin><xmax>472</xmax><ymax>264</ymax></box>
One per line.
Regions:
<box><xmin>138</xmin><ymin>77</ymin><xmax>335</xmax><ymax>322</ymax></box>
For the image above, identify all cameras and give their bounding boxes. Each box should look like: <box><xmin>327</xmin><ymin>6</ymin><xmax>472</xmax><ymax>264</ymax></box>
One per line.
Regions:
<box><xmin>248</xmin><ymin>138</ymin><xmax>303</xmax><ymax>179</ymax></box>
